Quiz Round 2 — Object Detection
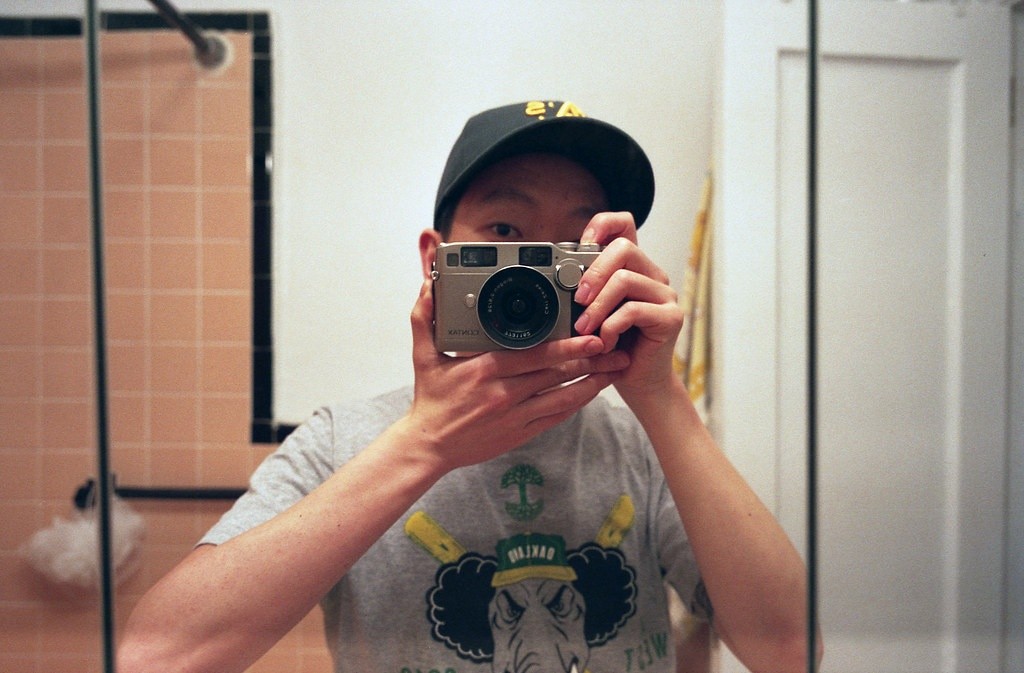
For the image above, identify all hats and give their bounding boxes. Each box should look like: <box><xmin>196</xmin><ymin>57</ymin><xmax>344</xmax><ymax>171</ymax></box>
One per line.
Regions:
<box><xmin>434</xmin><ymin>99</ymin><xmax>655</xmax><ymax>229</ymax></box>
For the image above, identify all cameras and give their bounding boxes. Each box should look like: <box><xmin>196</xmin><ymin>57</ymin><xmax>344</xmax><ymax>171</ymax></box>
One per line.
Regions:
<box><xmin>432</xmin><ymin>239</ymin><xmax>630</xmax><ymax>354</ymax></box>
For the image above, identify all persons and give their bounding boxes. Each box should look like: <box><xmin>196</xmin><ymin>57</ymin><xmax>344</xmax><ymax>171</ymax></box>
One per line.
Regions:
<box><xmin>115</xmin><ymin>100</ymin><xmax>825</xmax><ymax>673</ymax></box>
